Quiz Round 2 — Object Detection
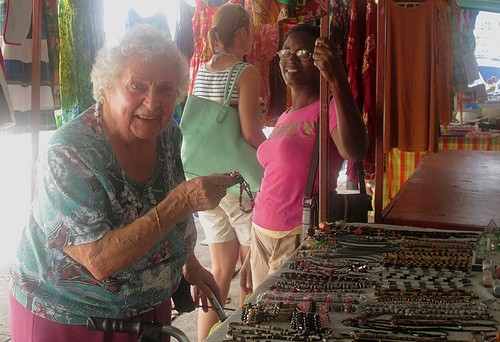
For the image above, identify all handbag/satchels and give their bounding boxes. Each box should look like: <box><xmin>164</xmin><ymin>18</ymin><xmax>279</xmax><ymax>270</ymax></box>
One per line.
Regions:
<box><xmin>177</xmin><ymin>59</ymin><xmax>265</xmax><ymax>197</ymax></box>
<box><xmin>311</xmin><ymin>194</ymin><xmax>373</xmax><ymax>228</ymax></box>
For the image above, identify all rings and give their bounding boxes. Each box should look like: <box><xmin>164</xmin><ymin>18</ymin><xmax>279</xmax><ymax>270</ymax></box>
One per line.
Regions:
<box><xmin>219</xmin><ymin>185</ymin><xmax>223</xmax><ymax>192</ymax></box>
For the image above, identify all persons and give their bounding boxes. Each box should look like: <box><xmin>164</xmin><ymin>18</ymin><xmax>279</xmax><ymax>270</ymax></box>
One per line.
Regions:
<box><xmin>240</xmin><ymin>24</ymin><xmax>366</xmax><ymax>294</ymax></box>
<box><xmin>8</xmin><ymin>28</ymin><xmax>239</xmax><ymax>342</ymax></box>
<box><xmin>192</xmin><ymin>3</ymin><xmax>267</xmax><ymax>342</ymax></box>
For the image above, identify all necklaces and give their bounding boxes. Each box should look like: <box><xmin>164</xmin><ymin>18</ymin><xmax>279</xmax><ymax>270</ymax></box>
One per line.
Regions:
<box><xmin>221</xmin><ymin>170</ymin><xmax>256</xmax><ymax>214</ymax></box>
<box><xmin>223</xmin><ymin>222</ymin><xmax>500</xmax><ymax>341</ymax></box>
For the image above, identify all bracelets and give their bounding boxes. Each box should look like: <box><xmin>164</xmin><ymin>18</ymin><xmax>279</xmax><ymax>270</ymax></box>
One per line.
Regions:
<box><xmin>154</xmin><ymin>206</ymin><xmax>162</xmax><ymax>234</ymax></box>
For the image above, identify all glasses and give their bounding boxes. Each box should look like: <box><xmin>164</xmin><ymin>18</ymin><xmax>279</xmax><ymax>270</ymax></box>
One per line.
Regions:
<box><xmin>277</xmin><ymin>50</ymin><xmax>313</xmax><ymax>61</ymax></box>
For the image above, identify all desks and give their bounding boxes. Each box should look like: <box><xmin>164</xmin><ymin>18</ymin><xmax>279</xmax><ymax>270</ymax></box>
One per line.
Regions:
<box><xmin>204</xmin><ymin>102</ymin><xmax>500</xmax><ymax>342</ymax></box>
